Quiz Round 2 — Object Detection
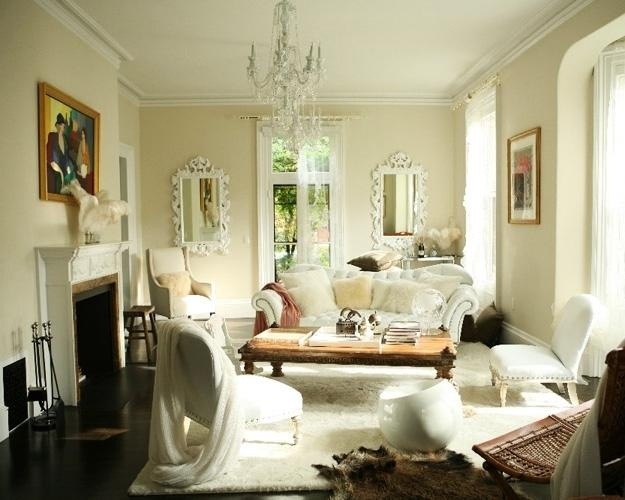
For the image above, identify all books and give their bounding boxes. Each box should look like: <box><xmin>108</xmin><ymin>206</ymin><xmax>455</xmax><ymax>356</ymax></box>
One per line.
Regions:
<box><xmin>382</xmin><ymin>321</ymin><xmax>421</xmax><ymax>345</ymax></box>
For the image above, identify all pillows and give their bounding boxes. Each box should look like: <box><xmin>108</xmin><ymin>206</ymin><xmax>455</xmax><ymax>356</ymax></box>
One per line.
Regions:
<box><xmin>474</xmin><ymin>300</ymin><xmax>504</xmax><ymax>346</ymax></box>
<box><xmin>282</xmin><ymin>266</ymin><xmax>462</xmax><ymax>317</ymax></box>
<box><xmin>347</xmin><ymin>250</ymin><xmax>406</xmax><ymax>270</ymax></box>
<box><xmin>153</xmin><ymin>272</ymin><xmax>194</xmax><ymax>295</ymax></box>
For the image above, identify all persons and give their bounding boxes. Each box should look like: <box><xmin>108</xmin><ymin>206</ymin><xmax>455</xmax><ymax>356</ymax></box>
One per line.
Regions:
<box><xmin>76</xmin><ymin>130</ymin><xmax>92</xmax><ymax>175</ymax></box>
<box><xmin>47</xmin><ymin>113</ymin><xmax>76</xmax><ymax>193</ymax></box>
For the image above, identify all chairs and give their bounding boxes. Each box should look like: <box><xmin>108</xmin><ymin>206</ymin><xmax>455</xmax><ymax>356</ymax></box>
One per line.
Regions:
<box><xmin>145</xmin><ymin>244</ymin><xmax>216</xmax><ymax>323</ymax></box>
<box><xmin>473</xmin><ymin>342</ymin><xmax>625</xmax><ymax>498</ymax></box>
<box><xmin>488</xmin><ymin>293</ymin><xmax>601</xmax><ymax>409</ymax></box>
<box><xmin>161</xmin><ymin>319</ymin><xmax>304</xmax><ymax>451</ymax></box>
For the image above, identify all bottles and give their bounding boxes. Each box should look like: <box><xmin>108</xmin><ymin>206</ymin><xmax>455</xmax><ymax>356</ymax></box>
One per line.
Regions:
<box><xmin>417</xmin><ymin>237</ymin><xmax>425</xmax><ymax>258</ymax></box>
<box><xmin>430</xmin><ymin>245</ymin><xmax>437</xmax><ymax>257</ymax></box>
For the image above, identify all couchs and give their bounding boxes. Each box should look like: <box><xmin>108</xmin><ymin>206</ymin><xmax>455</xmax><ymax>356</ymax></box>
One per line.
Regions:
<box><xmin>252</xmin><ymin>260</ymin><xmax>480</xmax><ymax>350</ymax></box>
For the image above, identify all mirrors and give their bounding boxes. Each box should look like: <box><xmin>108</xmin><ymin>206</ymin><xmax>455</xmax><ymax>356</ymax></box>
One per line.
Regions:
<box><xmin>370</xmin><ymin>151</ymin><xmax>427</xmax><ymax>251</ymax></box>
<box><xmin>168</xmin><ymin>153</ymin><xmax>230</xmax><ymax>256</ymax></box>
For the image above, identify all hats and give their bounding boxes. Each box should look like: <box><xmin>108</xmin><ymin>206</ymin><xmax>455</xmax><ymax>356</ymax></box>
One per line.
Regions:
<box><xmin>55</xmin><ymin>113</ymin><xmax>65</xmax><ymax>124</ymax></box>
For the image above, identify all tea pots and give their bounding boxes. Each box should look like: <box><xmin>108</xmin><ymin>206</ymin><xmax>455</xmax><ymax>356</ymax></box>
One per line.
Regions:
<box><xmin>358</xmin><ymin>320</ymin><xmax>376</xmax><ymax>339</ymax></box>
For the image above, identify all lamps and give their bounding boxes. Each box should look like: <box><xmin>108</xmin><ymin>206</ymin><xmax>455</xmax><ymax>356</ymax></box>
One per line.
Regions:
<box><xmin>245</xmin><ymin>0</ymin><xmax>323</xmax><ymax>155</ymax></box>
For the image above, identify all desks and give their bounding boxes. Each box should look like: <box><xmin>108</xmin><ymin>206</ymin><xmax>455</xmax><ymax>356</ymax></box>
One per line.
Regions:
<box><xmin>404</xmin><ymin>256</ymin><xmax>455</xmax><ymax>268</ymax></box>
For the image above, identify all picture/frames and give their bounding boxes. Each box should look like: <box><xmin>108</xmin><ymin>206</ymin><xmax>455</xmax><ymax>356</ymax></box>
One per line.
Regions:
<box><xmin>507</xmin><ymin>126</ymin><xmax>542</xmax><ymax>224</ymax></box>
<box><xmin>200</xmin><ymin>178</ymin><xmax>213</xmax><ymax>215</ymax></box>
<box><xmin>35</xmin><ymin>80</ymin><xmax>101</xmax><ymax>206</ymax></box>
<box><xmin>384</xmin><ymin>194</ymin><xmax>387</xmax><ymax>217</ymax></box>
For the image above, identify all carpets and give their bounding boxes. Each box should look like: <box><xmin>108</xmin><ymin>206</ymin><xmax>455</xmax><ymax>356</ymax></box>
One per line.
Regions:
<box><xmin>309</xmin><ymin>443</ymin><xmax>509</xmax><ymax>500</ymax></box>
<box><xmin>122</xmin><ymin>340</ymin><xmax>579</xmax><ymax>500</ymax></box>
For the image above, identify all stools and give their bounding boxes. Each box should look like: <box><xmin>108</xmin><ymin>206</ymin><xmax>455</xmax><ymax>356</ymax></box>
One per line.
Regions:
<box><xmin>123</xmin><ymin>305</ymin><xmax>157</xmax><ymax>366</ymax></box>
<box><xmin>380</xmin><ymin>377</ymin><xmax>463</xmax><ymax>453</ymax></box>
